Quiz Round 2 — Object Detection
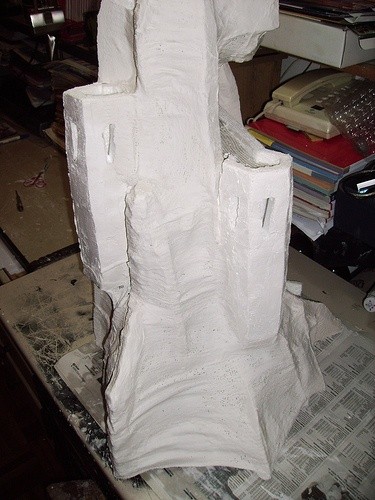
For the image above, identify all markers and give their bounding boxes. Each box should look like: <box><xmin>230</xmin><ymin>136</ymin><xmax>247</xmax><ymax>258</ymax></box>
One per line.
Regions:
<box><xmin>0</xmin><ymin>132</ymin><xmax>29</xmax><ymax>144</ymax></box>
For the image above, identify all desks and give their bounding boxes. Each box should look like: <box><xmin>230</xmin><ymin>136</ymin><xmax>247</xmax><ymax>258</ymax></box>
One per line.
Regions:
<box><xmin>1</xmin><ymin>108</ymin><xmax>374</xmax><ymax>498</ymax></box>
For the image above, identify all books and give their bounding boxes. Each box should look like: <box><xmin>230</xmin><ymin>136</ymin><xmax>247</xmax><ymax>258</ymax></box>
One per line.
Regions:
<box><xmin>0</xmin><ymin>21</ymin><xmax>96</xmax><ymax>152</ymax></box>
<box><xmin>248</xmin><ymin>118</ymin><xmax>375</xmax><ymax>244</ymax></box>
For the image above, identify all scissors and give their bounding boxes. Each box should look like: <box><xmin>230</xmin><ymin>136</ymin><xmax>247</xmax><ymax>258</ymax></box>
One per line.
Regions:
<box><xmin>23</xmin><ymin>171</ymin><xmax>47</xmax><ymax>189</ymax></box>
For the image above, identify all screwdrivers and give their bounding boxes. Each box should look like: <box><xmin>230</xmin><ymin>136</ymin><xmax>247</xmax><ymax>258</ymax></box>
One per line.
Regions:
<box><xmin>15</xmin><ymin>190</ymin><xmax>24</xmax><ymax>212</ymax></box>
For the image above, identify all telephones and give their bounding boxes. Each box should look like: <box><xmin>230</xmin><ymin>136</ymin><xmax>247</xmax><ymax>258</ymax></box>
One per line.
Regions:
<box><xmin>263</xmin><ymin>67</ymin><xmax>370</xmax><ymax>140</ymax></box>
